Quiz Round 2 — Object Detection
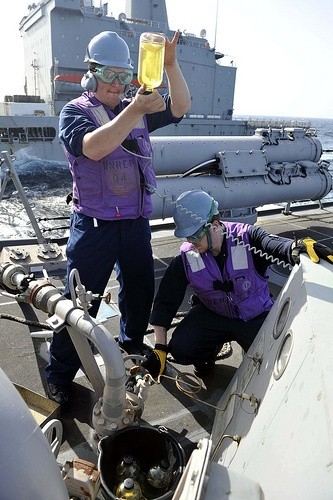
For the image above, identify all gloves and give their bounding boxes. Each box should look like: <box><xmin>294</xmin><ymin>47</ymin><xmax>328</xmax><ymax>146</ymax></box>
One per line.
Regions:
<box><xmin>152</xmin><ymin>343</ymin><xmax>168</xmax><ymax>380</ymax></box>
<box><xmin>291</xmin><ymin>236</ymin><xmax>333</xmax><ymax>265</ymax></box>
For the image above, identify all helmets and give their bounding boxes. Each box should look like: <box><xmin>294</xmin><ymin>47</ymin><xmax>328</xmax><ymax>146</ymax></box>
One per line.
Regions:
<box><xmin>174</xmin><ymin>189</ymin><xmax>218</xmax><ymax>238</ymax></box>
<box><xmin>83</xmin><ymin>31</ymin><xmax>134</xmax><ymax>69</ymax></box>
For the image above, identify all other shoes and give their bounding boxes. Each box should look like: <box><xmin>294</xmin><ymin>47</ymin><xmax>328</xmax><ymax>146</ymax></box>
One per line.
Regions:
<box><xmin>194</xmin><ymin>360</ymin><xmax>215</xmax><ymax>374</ymax></box>
<box><xmin>117</xmin><ymin>341</ymin><xmax>150</xmax><ymax>363</ymax></box>
<box><xmin>47</xmin><ymin>383</ymin><xmax>68</xmax><ymax>404</ymax></box>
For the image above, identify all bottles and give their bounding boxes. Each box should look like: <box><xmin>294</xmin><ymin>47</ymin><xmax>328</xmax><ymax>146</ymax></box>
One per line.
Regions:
<box><xmin>143</xmin><ymin>459</ymin><xmax>173</xmax><ymax>496</ymax></box>
<box><xmin>138</xmin><ymin>32</ymin><xmax>165</xmax><ymax>95</ymax></box>
<box><xmin>117</xmin><ymin>454</ymin><xmax>141</xmax><ymax>482</ymax></box>
<box><xmin>116</xmin><ymin>477</ymin><xmax>144</xmax><ymax>500</ymax></box>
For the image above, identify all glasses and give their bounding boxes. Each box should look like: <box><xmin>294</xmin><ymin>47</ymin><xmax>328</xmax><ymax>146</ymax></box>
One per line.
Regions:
<box><xmin>95</xmin><ymin>66</ymin><xmax>133</xmax><ymax>85</ymax></box>
<box><xmin>183</xmin><ymin>236</ymin><xmax>200</xmax><ymax>244</ymax></box>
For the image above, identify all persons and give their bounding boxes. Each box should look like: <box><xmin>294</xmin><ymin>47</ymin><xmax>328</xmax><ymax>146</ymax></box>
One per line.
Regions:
<box><xmin>141</xmin><ymin>190</ymin><xmax>333</xmax><ymax>379</ymax></box>
<box><xmin>44</xmin><ymin>29</ymin><xmax>192</xmax><ymax>406</ymax></box>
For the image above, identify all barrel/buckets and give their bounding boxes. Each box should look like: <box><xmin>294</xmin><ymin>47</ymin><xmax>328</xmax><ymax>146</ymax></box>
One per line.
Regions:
<box><xmin>94</xmin><ymin>424</ymin><xmax>186</xmax><ymax>500</ymax></box>
